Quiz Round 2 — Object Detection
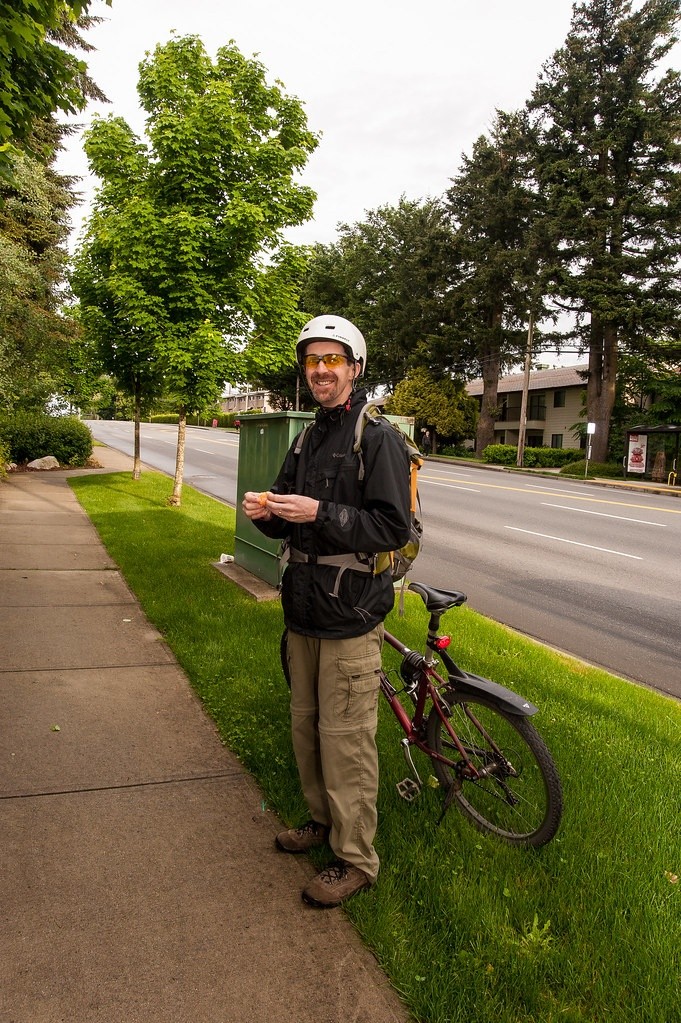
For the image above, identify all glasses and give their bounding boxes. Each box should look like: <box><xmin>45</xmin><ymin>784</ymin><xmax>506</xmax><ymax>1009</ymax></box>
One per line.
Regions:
<box><xmin>303</xmin><ymin>353</ymin><xmax>356</xmax><ymax>367</ymax></box>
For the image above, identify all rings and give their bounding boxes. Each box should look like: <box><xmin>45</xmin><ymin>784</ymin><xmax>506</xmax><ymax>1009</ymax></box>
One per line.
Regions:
<box><xmin>278</xmin><ymin>509</ymin><xmax>283</xmax><ymax>515</ymax></box>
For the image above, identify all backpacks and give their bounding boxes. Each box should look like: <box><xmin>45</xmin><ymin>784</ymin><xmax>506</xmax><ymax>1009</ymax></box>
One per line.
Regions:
<box><xmin>279</xmin><ymin>404</ymin><xmax>423</xmax><ymax>582</ymax></box>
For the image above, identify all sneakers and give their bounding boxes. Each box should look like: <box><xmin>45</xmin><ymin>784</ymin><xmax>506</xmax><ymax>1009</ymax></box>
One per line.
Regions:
<box><xmin>302</xmin><ymin>857</ymin><xmax>372</xmax><ymax>910</ymax></box>
<box><xmin>275</xmin><ymin>819</ymin><xmax>330</xmax><ymax>855</ymax></box>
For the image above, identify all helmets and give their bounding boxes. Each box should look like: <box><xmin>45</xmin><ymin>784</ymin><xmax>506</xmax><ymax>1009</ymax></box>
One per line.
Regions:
<box><xmin>296</xmin><ymin>315</ymin><xmax>367</xmax><ymax>380</ymax></box>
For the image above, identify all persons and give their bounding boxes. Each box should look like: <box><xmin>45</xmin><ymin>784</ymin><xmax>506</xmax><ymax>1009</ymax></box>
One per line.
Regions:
<box><xmin>242</xmin><ymin>314</ymin><xmax>412</xmax><ymax>911</ymax></box>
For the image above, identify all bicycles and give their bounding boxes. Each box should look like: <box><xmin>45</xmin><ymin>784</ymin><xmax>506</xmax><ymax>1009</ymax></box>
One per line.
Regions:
<box><xmin>282</xmin><ymin>582</ymin><xmax>563</xmax><ymax>853</ymax></box>
